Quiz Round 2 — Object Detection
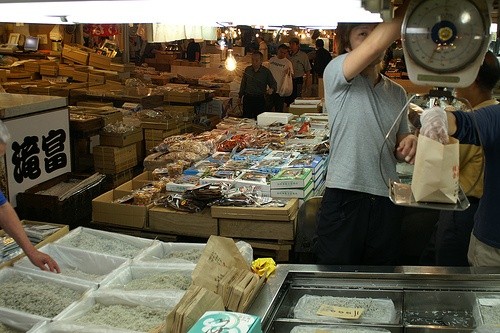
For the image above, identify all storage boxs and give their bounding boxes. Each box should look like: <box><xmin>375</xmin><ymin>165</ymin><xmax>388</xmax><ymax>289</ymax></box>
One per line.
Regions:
<box><xmin>0</xmin><ymin>31</ymin><xmax>436</xmax><ymax>333</ymax></box>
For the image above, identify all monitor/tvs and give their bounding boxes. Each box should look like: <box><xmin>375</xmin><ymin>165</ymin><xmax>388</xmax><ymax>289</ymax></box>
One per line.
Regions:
<box><xmin>24</xmin><ymin>37</ymin><xmax>40</xmax><ymax>51</ymax></box>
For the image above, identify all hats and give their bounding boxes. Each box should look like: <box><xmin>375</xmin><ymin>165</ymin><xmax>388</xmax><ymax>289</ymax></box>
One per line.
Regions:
<box><xmin>316</xmin><ymin>39</ymin><xmax>324</xmax><ymax>47</ymax></box>
<box><xmin>256</xmin><ymin>32</ymin><xmax>265</xmax><ymax>40</ymax></box>
<box><xmin>288</xmin><ymin>38</ymin><xmax>299</xmax><ymax>45</ymax></box>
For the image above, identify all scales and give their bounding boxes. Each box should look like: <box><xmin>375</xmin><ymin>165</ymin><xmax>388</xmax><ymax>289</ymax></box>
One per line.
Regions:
<box><xmin>379</xmin><ymin>0</ymin><xmax>492</xmax><ymax>211</ymax></box>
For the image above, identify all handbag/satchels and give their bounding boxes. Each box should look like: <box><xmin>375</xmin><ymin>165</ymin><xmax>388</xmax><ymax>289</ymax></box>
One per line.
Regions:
<box><xmin>278</xmin><ymin>66</ymin><xmax>293</xmax><ymax>96</ymax></box>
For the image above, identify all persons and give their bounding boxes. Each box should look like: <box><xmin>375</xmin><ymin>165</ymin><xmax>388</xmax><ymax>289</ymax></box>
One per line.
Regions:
<box><xmin>437</xmin><ymin>50</ymin><xmax>500</xmax><ymax>266</ymax></box>
<box><xmin>445</xmin><ymin>103</ymin><xmax>500</xmax><ymax>266</ymax></box>
<box><xmin>314</xmin><ymin>39</ymin><xmax>332</xmax><ymax>98</ymax></box>
<box><xmin>258</xmin><ymin>35</ymin><xmax>268</xmax><ymax>62</ymax></box>
<box><xmin>266</xmin><ymin>43</ymin><xmax>293</xmax><ymax>113</ymax></box>
<box><xmin>238</xmin><ymin>52</ymin><xmax>278</xmax><ymax>120</ymax></box>
<box><xmin>0</xmin><ymin>191</ymin><xmax>61</xmax><ymax>273</ymax></box>
<box><xmin>311</xmin><ymin>14</ymin><xmax>419</xmax><ymax>265</ymax></box>
<box><xmin>287</xmin><ymin>38</ymin><xmax>311</xmax><ymax>103</ymax></box>
<box><xmin>187</xmin><ymin>39</ymin><xmax>201</xmax><ymax>62</ymax></box>
